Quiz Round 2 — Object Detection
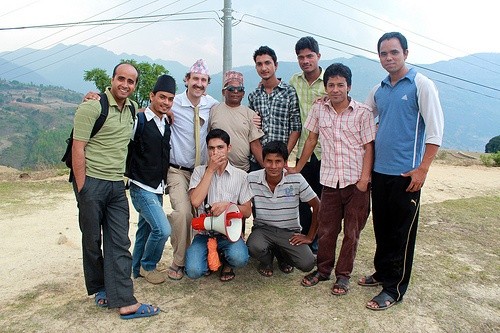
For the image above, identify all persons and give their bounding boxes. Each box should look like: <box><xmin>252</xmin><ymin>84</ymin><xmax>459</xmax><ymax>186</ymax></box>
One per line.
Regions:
<box><xmin>246</xmin><ymin>46</ymin><xmax>302</xmax><ymax>218</ymax></box>
<box><xmin>83</xmin><ymin>74</ymin><xmax>176</xmax><ymax>284</ymax></box>
<box><xmin>209</xmin><ymin>71</ymin><xmax>263</xmax><ymax>235</ymax></box>
<box><xmin>284</xmin><ymin>61</ymin><xmax>375</xmax><ymax>296</ymax></box>
<box><xmin>185</xmin><ymin>129</ymin><xmax>255</xmax><ymax>283</ymax></box>
<box><xmin>73</xmin><ymin>62</ymin><xmax>174</xmax><ymax>320</ymax></box>
<box><xmin>246</xmin><ymin>139</ymin><xmax>315</xmax><ymax>278</ymax></box>
<box><xmin>310</xmin><ymin>31</ymin><xmax>444</xmax><ymax>310</ymax></box>
<box><xmin>168</xmin><ymin>60</ymin><xmax>261</xmax><ymax>280</ymax></box>
<box><xmin>257</xmin><ymin>36</ymin><xmax>329</xmax><ymax>255</ymax></box>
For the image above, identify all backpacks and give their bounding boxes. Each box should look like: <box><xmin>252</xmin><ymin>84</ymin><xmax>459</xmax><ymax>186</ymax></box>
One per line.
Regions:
<box><xmin>61</xmin><ymin>93</ymin><xmax>135</xmax><ymax>183</ymax></box>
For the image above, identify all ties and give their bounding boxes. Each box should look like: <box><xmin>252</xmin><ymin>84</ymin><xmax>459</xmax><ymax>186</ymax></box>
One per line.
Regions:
<box><xmin>194</xmin><ymin>107</ymin><xmax>200</xmax><ymax>167</ymax></box>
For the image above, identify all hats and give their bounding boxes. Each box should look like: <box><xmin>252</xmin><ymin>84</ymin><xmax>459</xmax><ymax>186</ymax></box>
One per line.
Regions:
<box><xmin>187</xmin><ymin>59</ymin><xmax>210</xmax><ymax>77</ymax></box>
<box><xmin>153</xmin><ymin>75</ymin><xmax>175</xmax><ymax>95</ymax></box>
<box><xmin>223</xmin><ymin>71</ymin><xmax>244</xmax><ymax>87</ymax></box>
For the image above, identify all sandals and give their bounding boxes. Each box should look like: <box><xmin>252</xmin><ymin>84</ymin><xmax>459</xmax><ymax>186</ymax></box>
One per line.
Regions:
<box><xmin>366</xmin><ymin>290</ymin><xmax>398</xmax><ymax>310</ymax></box>
<box><xmin>357</xmin><ymin>272</ymin><xmax>384</xmax><ymax>286</ymax></box>
<box><xmin>301</xmin><ymin>271</ymin><xmax>330</xmax><ymax>287</ymax></box>
<box><xmin>279</xmin><ymin>262</ymin><xmax>294</xmax><ymax>273</ymax></box>
<box><xmin>220</xmin><ymin>262</ymin><xmax>235</xmax><ymax>282</ymax></box>
<box><xmin>257</xmin><ymin>261</ymin><xmax>273</xmax><ymax>277</ymax></box>
<box><xmin>331</xmin><ymin>278</ymin><xmax>350</xmax><ymax>295</ymax></box>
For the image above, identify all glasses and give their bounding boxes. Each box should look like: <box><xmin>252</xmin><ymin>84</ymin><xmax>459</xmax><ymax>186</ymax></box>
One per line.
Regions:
<box><xmin>114</xmin><ymin>74</ymin><xmax>137</xmax><ymax>85</ymax></box>
<box><xmin>224</xmin><ymin>86</ymin><xmax>245</xmax><ymax>92</ymax></box>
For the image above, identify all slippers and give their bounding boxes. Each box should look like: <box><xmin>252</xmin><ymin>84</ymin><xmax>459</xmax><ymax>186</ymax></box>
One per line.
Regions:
<box><xmin>168</xmin><ymin>262</ymin><xmax>184</xmax><ymax>280</ymax></box>
<box><xmin>120</xmin><ymin>303</ymin><xmax>160</xmax><ymax>319</ymax></box>
<box><xmin>95</xmin><ymin>289</ymin><xmax>108</xmax><ymax>307</ymax></box>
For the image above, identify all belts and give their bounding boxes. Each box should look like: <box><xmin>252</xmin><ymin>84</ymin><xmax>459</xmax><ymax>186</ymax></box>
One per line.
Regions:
<box><xmin>169</xmin><ymin>163</ymin><xmax>194</xmax><ymax>172</ymax></box>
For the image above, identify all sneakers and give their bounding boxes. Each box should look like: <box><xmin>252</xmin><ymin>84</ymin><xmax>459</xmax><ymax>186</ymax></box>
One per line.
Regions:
<box><xmin>140</xmin><ymin>263</ymin><xmax>165</xmax><ymax>283</ymax></box>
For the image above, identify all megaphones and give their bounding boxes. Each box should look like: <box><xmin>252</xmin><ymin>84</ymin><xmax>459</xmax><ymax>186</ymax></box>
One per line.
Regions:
<box><xmin>191</xmin><ymin>202</ymin><xmax>243</xmax><ymax>243</ymax></box>
